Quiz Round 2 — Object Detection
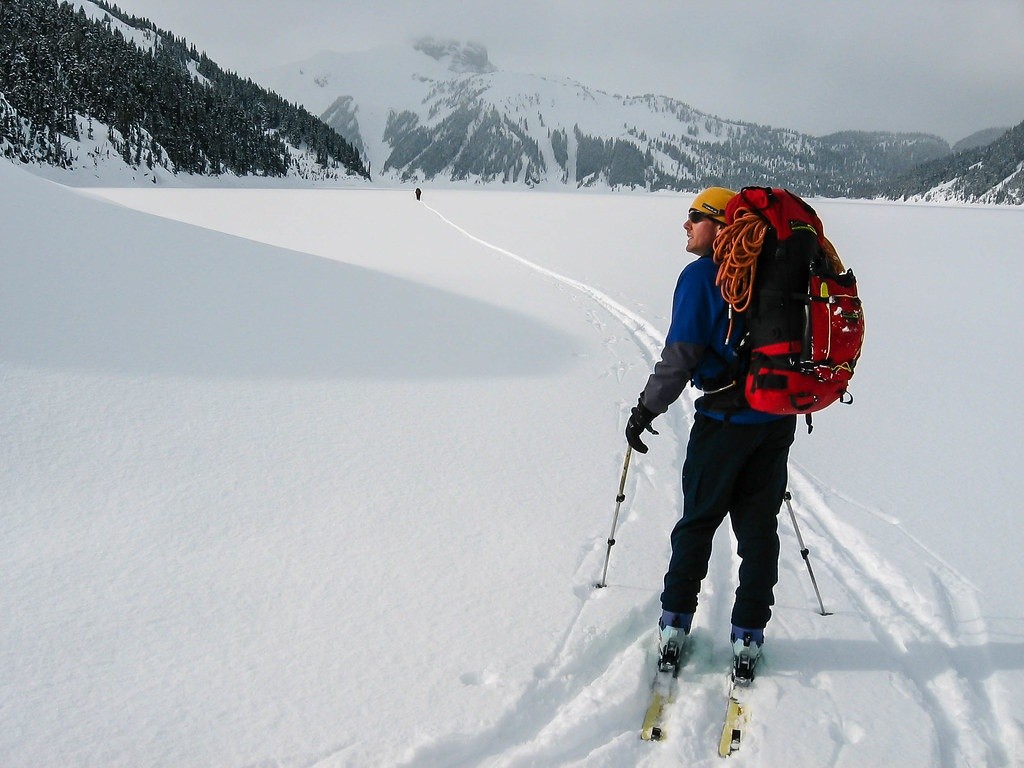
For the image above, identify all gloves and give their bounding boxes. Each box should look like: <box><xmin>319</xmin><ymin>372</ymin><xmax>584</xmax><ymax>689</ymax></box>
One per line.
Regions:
<box><xmin>626</xmin><ymin>398</ymin><xmax>658</xmax><ymax>453</ymax></box>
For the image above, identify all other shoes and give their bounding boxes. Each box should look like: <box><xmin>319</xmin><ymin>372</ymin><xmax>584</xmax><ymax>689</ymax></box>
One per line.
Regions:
<box><xmin>658</xmin><ymin>610</ymin><xmax>694</xmax><ymax>677</ymax></box>
<box><xmin>731</xmin><ymin>625</ymin><xmax>764</xmax><ymax>687</ymax></box>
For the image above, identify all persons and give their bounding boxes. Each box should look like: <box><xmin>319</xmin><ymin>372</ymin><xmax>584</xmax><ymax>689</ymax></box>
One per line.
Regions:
<box><xmin>415</xmin><ymin>188</ymin><xmax>422</xmax><ymax>201</ymax></box>
<box><xmin>625</xmin><ymin>187</ymin><xmax>796</xmax><ymax>687</ymax></box>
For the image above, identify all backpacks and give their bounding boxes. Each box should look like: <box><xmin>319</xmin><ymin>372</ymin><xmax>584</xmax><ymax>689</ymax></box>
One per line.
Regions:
<box><xmin>702</xmin><ymin>188</ymin><xmax>864</xmax><ymax>435</ymax></box>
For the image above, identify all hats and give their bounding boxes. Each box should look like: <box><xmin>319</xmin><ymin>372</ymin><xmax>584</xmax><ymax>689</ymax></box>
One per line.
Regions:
<box><xmin>690</xmin><ymin>187</ymin><xmax>736</xmax><ymax>225</ymax></box>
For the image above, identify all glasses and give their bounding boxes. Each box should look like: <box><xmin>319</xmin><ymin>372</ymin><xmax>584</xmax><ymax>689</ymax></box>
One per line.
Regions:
<box><xmin>688</xmin><ymin>212</ymin><xmax>709</xmax><ymax>224</ymax></box>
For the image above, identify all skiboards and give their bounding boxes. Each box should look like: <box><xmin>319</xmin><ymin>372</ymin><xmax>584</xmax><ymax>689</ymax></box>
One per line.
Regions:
<box><xmin>718</xmin><ymin>683</ymin><xmax>754</xmax><ymax>758</ymax></box>
<box><xmin>641</xmin><ymin>672</ymin><xmax>678</xmax><ymax>743</ymax></box>
